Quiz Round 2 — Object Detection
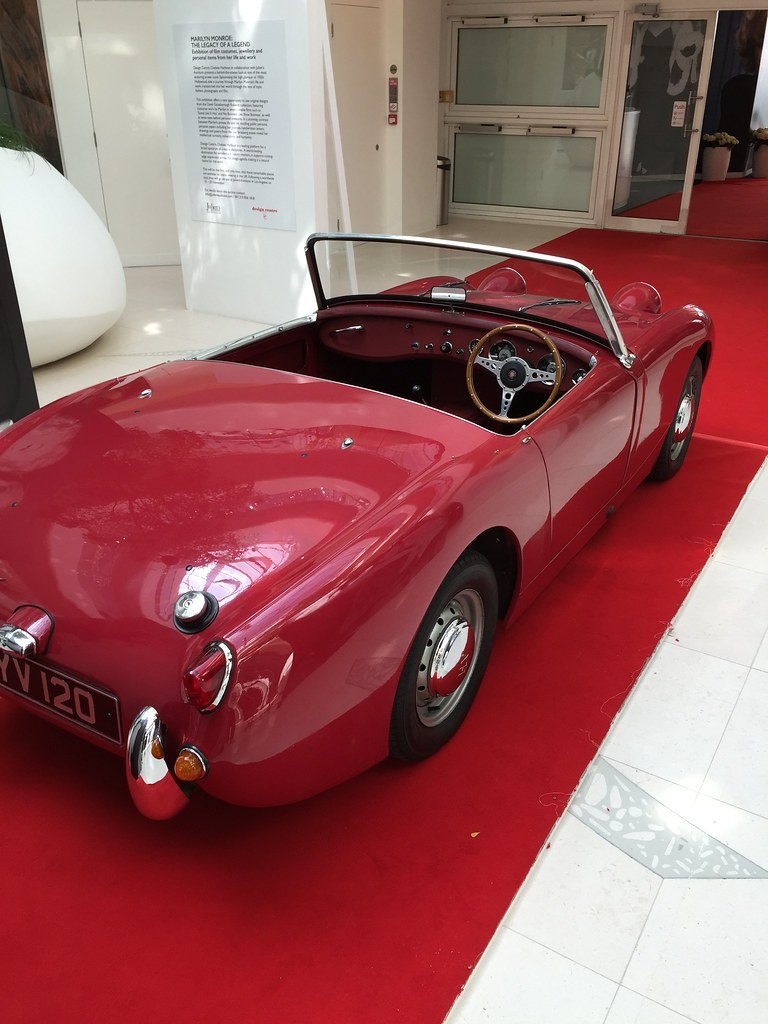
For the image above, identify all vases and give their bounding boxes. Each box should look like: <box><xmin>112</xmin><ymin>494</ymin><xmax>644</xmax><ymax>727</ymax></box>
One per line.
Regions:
<box><xmin>701</xmin><ymin>146</ymin><xmax>732</xmax><ymax>181</ymax></box>
<box><xmin>751</xmin><ymin>143</ymin><xmax>768</xmax><ymax>178</ymax></box>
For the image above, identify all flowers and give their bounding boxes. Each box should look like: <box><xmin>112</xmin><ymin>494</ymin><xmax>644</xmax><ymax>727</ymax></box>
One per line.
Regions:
<box><xmin>700</xmin><ymin>132</ymin><xmax>740</xmax><ymax>149</ymax></box>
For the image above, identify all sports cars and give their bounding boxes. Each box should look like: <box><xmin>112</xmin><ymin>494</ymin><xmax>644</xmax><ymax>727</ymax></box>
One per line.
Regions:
<box><xmin>0</xmin><ymin>233</ymin><xmax>713</xmax><ymax>822</ymax></box>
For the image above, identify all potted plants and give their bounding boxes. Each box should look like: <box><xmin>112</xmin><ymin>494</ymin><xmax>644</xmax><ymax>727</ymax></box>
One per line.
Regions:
<box><xmin>748</xmin><ymin>127</ymin><xmax>768</xmax><ymax>145</ymax></box>
